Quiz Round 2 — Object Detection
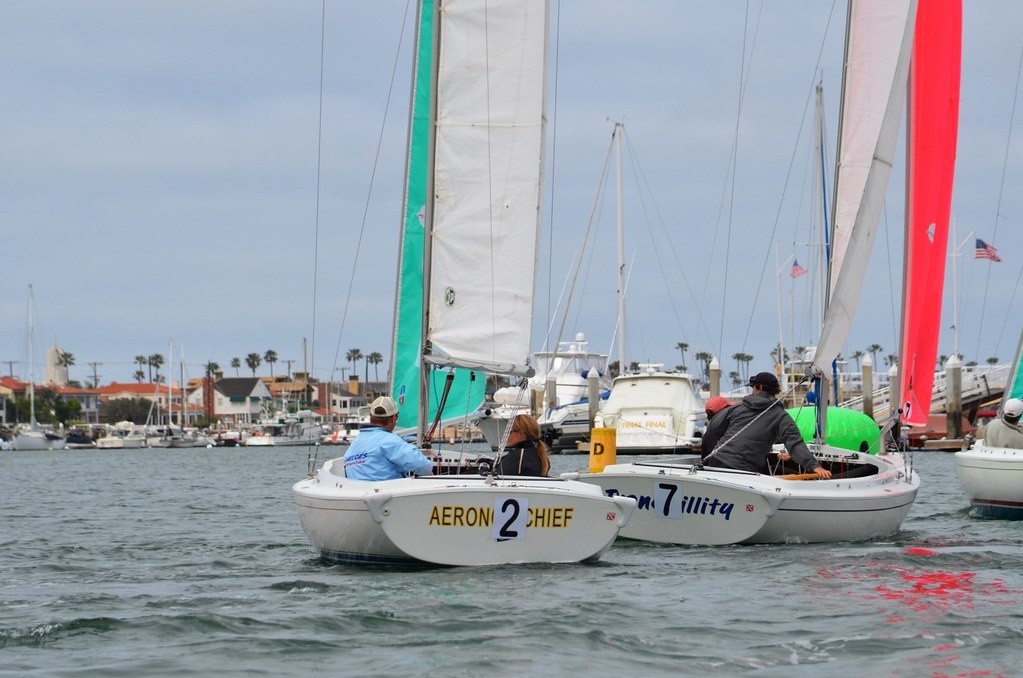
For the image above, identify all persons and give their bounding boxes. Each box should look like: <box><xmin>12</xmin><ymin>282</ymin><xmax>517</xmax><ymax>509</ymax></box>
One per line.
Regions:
<box><xmin>701</xmin><ymin>372</ymin><xmax>832</xmax><ymax>478</ymax></box>
<box><xmin>494</xmin><ymin>414</ymin><xmax>551</xmax><ymax>476</ymax></box>
<box><xmin>705</xmin><ymin>397</ymin><xmax>807</xmax><ymax>474</ymax></box>
<box><xmin>343</xmin><ymin>396</ymin><xmax>433</xmax><ymax>481</ymax></box>
<box><xmin>253</xmin><ymin>430</ymin><xmax>260</xmax><ymax>436</ymax></box>
<box><xmin>983</xmin><ymin>397</ymin><xmax>1023</xmax><ymax>449</ymax></box>
<box><xmin>264</xmin><ymin>430</ymin><xmax>271</xmax><ymax>436</ymax></box>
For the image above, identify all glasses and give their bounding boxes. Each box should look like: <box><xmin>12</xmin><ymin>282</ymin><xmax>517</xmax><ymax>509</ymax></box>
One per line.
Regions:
<box><xmin>750</xmin><ymin>377</ymin><xmax>759</xmax><ymax>384</ymax></box>
<box><xmin>508</xmin><ymin>430</ymin><xmax>519</xmax><ymax>434</ymax></box>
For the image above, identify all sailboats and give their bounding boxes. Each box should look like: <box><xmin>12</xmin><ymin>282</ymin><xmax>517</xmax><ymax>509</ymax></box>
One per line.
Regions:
<box><xmin>288</xmin><ymin>0</ymin><xmax>1023</xmax><ymax>567</ymax></box>
<box><xmin>0</xmin><ymin>284</ymin><xmax>67</xmax><ymax>450</ymax></box>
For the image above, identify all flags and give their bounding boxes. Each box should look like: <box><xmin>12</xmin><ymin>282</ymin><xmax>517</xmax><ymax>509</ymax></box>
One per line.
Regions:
<box><xmin>790</xmin><ymin>259</ymin><xmax>807</xmax><ymax>278</ymax></box>
<box><xmin>975</xmin><ymin>238</ymin><xmax>1001</xmax><ymax>262</ymax></box>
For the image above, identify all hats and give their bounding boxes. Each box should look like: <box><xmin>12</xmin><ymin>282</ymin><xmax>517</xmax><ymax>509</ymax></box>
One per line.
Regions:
<box><xmin>706</xmin><ymin>397</ymin><xmax>736</xmax><ymax>414</ymax></box>
<box><xmin>745</xmin><ymin>373</ymin><xmax>779</xmax><ymax>393</ymax></box>
<box><xmin>370</xmin><ymin>396</ymin><xmax>399</xmax><ymax>417</ymax></box>
<box><xmin>1004</xmin><ymin>398</ymin><xmax>1023</xmax><ymax>417</ymax></box>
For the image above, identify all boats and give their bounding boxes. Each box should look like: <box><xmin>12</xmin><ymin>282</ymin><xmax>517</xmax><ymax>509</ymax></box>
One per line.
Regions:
<box><xmin>66</xmin><ymin>340</ymin><xmax>372</xmax><ymax>448</ymax></box>
<box><xmin>592</xmin><ymin>364</ymin><xmax>707</xmax><ymax>449</ymax></box>
